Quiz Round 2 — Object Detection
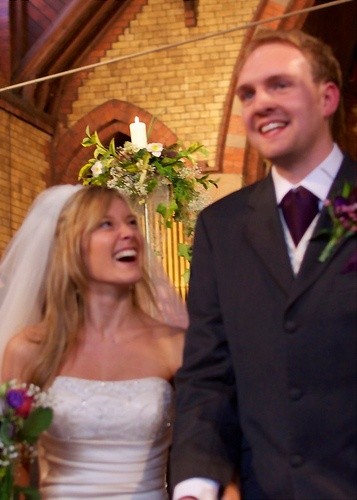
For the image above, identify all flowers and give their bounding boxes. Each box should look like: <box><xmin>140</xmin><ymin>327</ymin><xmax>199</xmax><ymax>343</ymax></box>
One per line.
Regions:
<box><xmin>0</xmin><ymin>380</ymin><xmax>55</xmax><ymax>500</ymax></box>
<box><xmin>76</xmin><ymin>115</ymin><xmax>220</xmax><ymax>284</ymax></box>
<box><xmin>318</xmin><ymin>182</ymin><xmax>357</xmax><ymax>260</ymax></box>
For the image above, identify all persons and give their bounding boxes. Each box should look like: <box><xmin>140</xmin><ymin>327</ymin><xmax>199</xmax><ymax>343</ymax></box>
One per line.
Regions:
<box><xmin>164</xmin><ymin>28</ymin><xmax>357</xmax><ymax>500</ymax></box>
<box><xmin>0</xmin><ymin>183</ymin><xmax>243</xmax><ymax>500</ymax></box>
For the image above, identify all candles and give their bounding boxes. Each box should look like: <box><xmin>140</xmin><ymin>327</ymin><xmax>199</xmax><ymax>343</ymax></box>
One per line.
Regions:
<box><xmin>129</xmin><ymin>117</ymin><xmax>148</xmax><ymax>146</ymax></box>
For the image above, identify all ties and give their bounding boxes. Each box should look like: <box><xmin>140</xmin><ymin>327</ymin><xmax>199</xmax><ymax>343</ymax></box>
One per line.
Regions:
<box><xmin>281</xmin><ymin>186</ymin><xmax>319</xmax><ymax>247</ymax></box>
